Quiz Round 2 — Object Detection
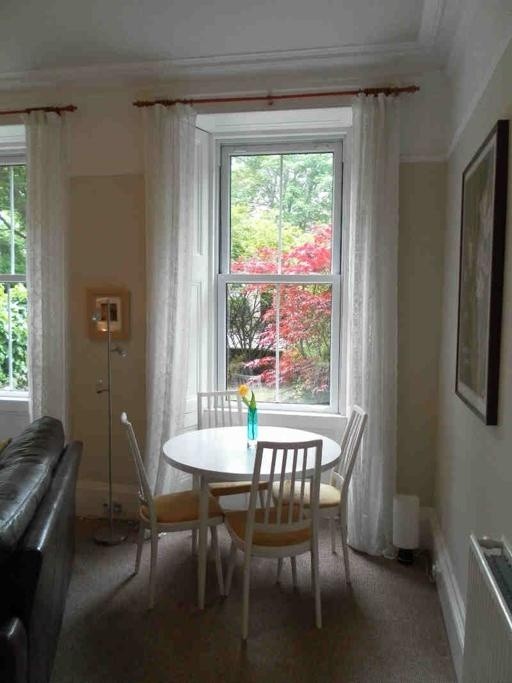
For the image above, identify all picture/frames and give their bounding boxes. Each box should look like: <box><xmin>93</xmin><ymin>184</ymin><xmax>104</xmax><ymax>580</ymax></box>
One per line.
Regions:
<box><xmin>454</xmin><ymin>119</ymin><xmax>510</xmax><ymax>426</ymax></box>
<box><xmin>86</xmin><ymin>287</ymin><xmax>131</xmax><ymax>340</ymax></box>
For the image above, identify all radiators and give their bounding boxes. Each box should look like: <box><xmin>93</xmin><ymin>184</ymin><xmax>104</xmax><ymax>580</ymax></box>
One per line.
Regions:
<box><xmin>459</xmin><ymin>532</ymin><xmax>512</xmax><ymax>683</ymax></box>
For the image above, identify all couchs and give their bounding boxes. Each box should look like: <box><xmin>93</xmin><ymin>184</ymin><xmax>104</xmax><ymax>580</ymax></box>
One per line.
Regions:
<box><xmin>1</xmin><ymin>415</ymin><xmax>86</xmax><ymax>683</ymax></box>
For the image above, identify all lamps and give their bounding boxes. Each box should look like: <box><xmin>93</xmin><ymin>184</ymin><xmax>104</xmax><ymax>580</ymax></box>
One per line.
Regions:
<box><xmin>91</xmin><ymin>298</ymin><xmax>130</xmax><ymax>546</ymax></box>
<box><xmin>393</xmin><ymin>494</ymin><xmax>419</xmax><ymax>566</ymax></box>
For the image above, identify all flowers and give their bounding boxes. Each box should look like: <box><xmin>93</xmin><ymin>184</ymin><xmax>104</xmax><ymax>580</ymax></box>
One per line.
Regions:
<box><xmin>239</xmin><ymin>384</ymin><xmax>257</xmax><ymax>440</ymax></box>
